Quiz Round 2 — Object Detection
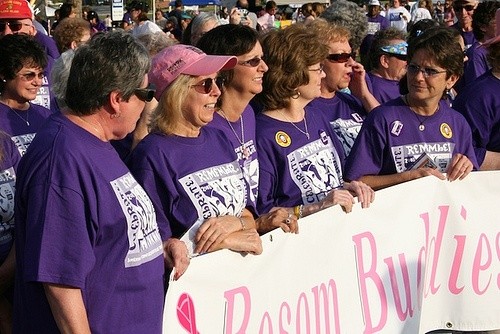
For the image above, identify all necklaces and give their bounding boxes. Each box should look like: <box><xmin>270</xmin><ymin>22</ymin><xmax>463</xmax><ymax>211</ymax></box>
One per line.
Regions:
<box><xmin>11</xmin><ymin>107</ymin><xmax>30</xmax><ymax>127</ymax></box>
<box><xmin>220</xmin><ymin>109</ymin><xmax>250</xmax><ymax>159</ymax></box>
<box><xmin>276</xmin><ymin>109</ymin><xmax>310</xmax><ymax>140</ymax></box>
<box><xmin>405</xmin><ymin>95</ymin><xmax>432</xmax><ymax>132</ymax></box>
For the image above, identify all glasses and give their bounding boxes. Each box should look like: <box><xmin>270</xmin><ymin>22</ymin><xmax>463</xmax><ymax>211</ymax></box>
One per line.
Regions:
<box><xmin>194</xmin><ymin>32</ymin><xmax>205</xmax><ymax>37</ymax></box>
<box><xmin>380</xmin><ymin>53</ymin><xmax>408</xmax><ymax>61</ymax></box>
<box><xmin>15</xmin><ymin>70</ymin><xmax>48</xmax><ymax>81</ymax></box>
<box><xmin>324</xmin><ymin>52</ymin><xmax>356</xmax><ymax>63</ymax></box>
<box><xmin>132</xmin><ymin>86</ymin><xmax>156</xmax><ymax>102</ymax></box>
<box><xmin>453</xmin><ymin>4</ymin><xmax>476</xmax><ymax>12</ymax></box>
<box><xmin>188</xmin><ymin>77</ymin><xmax>227</xmax><ymax>94</ymax></box>
<box><xmin>406</xmin><ymin>63</ymin><xmax>447</xmax><ymax>77</ymax></box>
<box><xmin>0</xmin><ymin>20</ymin><xmax>32</xmax><ymax>31</ymax></box>
<box><xmin>308</xmin><ymin>65</ymin><xmax>323</xmax><ymax>74</ymax></box>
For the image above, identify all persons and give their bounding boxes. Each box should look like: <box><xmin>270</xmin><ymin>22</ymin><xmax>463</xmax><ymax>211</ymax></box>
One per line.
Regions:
<box><xmin>256</xmin><ymin>23</ymin><xmax>375</xmax><ymax>218</ymax></box>
<box><xmin>12</xmin><ymin>29</ymin><xmax>189</xmax><ymax>334</ymax></box>
<box><xmin>345</xmin><ymin>26</ymin><xmax>479</xmax><ymax>191</ymax></box>
<box><xmin>0</xmin><ymin>0</ymin><xmax>500</xmax><ymax>334</ymax></box>
<box><xmin>128</xmin><ymin>45</ymin><xmax>264</xmax><ymax>255</ymax></box>
<box><xmin>197</xmin><ymin>25</ymin><xmax>300</xmax><ymax>233</ymax></box>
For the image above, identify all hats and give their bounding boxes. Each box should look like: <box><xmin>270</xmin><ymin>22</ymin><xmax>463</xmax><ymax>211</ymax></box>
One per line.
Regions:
<box><xmin>0</xmin><ymin>0</ymin><xmax>34</xmax><ymax>21</ymax></box>
<box><xmin>369</xmin><ymin>0</ymin><xmax>380</xmax><ymax>6</ymax></box>
<box><xmin>147</xmin><ymin>44</ymin><xmax>238</xmax><ymax>101</ymax></box>
<box><xmin>378</xmin><ymin>42</ymin><xmax>408</xmax><ymax>55</ymax></box>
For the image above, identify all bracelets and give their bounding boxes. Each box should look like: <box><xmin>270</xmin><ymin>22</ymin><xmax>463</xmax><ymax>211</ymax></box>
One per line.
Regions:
<box><xmin>240</xmin><ymin>216</ymin><xmax>245</xmax><ymax>232</ymax></box>
<box><xmin>295</xmin><ymin>205</ymin><xmax>299</xmax><ymax>220</ymax></box>
<box><xmin>299</xmin><ymin>204</ymin><xmax>303</xmax><ymax>217</ymax></box>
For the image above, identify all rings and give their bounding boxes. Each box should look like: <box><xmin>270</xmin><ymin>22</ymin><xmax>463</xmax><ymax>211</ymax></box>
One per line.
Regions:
<box><xmin>288</xmin><ymin>213</ymin><xmax>292</xmax><ymax>217</ymax></box>
<box><xmin>285</xmin><ymin>219</ymin><xmax>290</xmax><ymax>224</ymax></box>
